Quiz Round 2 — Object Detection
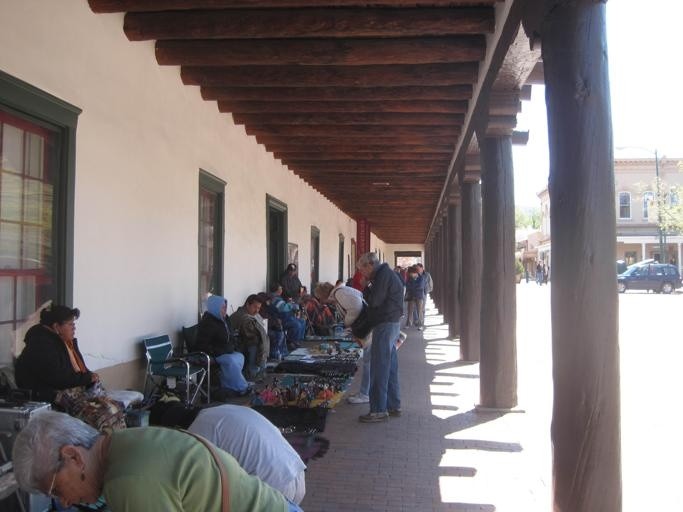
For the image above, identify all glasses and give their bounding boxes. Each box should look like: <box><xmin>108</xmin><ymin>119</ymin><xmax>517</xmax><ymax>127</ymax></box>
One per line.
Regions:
<box><xmin>43</xmin><ymin>468</ymin><xmax>59</xmax><ymax>499</ymax></box>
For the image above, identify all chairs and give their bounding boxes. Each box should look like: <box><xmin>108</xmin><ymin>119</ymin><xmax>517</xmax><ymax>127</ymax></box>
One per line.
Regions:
<box><xmin>182</xmin><ymin>325</ymin><xmax>198</xmax><ymax>348</ymax></box>
<box><xmin>141</xmin><ymin>335</ymin><xmax>212</xmax><ymax>417</ymax></box>
<box><xmin>0</xmin><ymin>359</ymin><xmax>144</xmax><ymax>511</ymax></box>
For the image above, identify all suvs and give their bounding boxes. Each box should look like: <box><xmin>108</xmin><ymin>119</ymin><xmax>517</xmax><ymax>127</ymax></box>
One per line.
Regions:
<box><xmin>616</xmin><ymin>262</ymin><xmax>682</xmax><ymax>295</ymax></box>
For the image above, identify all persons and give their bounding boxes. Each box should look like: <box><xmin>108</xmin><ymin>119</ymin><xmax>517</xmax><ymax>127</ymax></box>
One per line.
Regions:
<box><xmin>524</xmin><ymin>260</ymin><xmax>549</xmax><ymax>285</ymax></box>
<box><xmin>355</xmin><ymin>253</ymin><xmax>405</xmax><ymax>421</ymax></box>
<box><xmin>195</xmin><ymin>264</ymin><xmax>436</xmax><ymax>403</ymax></box>
<box><xmin>16</xmin><ymin>303</ymin><xmax>126</xmax><ymax>432</ymax></box>
<box><xmin>8</xmin><ymin>409</ymin><xmax>304</xmax><ymax>511</ymax></box>
<box><xmin>149</xmin><ymin>400</ymin><xmax>307</xmax><ymax>505</ymax></box>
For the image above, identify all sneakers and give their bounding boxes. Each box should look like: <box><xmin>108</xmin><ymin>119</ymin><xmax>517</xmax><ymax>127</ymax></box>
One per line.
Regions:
<box><xmin>404</xmin><ymin>326</ymin><xmax>423</xmax><ymax>330</ymax></box>
<box><xmin>348</xmin><ymin>392</ymin><xmax>369</xmax><ymax>404</ymax></box>
<box><xmin>388</xmin><ymin>408</ymin><xmax>403</xmax><ymax>416</ymax></box>
<box><xmin>359</xmin><ymin>411</ymin><xmax>389</xmax><ymax>422</ymax></box>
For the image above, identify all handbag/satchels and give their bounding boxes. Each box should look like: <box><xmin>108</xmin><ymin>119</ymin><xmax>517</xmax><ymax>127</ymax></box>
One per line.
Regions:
<box><xmin>150</xmin><ymin>399</ymin><xmax>188</xmax><ymax>426</ymax></box>
<box><xmin>351</xmin><ymin>304</ymin><xmax>372</xmax><ymax>338</ymax></box>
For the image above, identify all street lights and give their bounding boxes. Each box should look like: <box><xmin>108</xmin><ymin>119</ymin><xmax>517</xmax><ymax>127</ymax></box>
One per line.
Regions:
<box><xmin>616</xmin><ymin>143</ymin><xmax>665</xmax><ymax>263</ymax></box>
